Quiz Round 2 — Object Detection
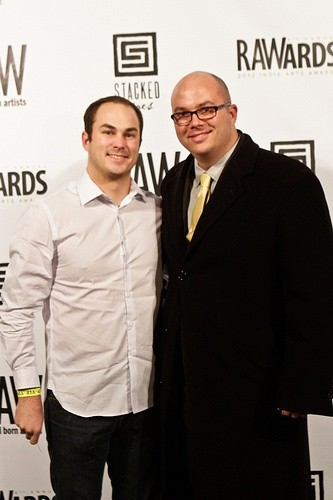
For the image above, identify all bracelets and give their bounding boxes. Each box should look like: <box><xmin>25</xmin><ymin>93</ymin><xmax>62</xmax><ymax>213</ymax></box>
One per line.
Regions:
<box><xmin>17</xmin><ymin>387</ymin><xmax>41</xmax><ymax>398</ymax></box>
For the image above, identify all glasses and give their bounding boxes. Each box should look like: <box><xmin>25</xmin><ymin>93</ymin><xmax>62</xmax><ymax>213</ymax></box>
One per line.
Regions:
<box><xmin>171</xmin><ymin>102</ymin><xmax>229</xmax><ymax>126</ymax></box>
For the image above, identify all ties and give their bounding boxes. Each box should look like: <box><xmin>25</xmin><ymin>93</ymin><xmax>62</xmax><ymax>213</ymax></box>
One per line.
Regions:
<box><xmin>186</xmin><ymin>174</ymin><xmax>211</xmax><ymax>241</ymax></box>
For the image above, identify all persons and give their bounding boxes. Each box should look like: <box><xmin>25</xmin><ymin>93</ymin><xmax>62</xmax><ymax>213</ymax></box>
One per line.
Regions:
<box><xmin>1</xmin><ymin>95</ymin><xmax>161</xmax><ymax>500</ymax></box>
<box><xmin>154</xmin><ymin>70</ymin><xmax>333</xmax><ymax>500</ymax></box>
